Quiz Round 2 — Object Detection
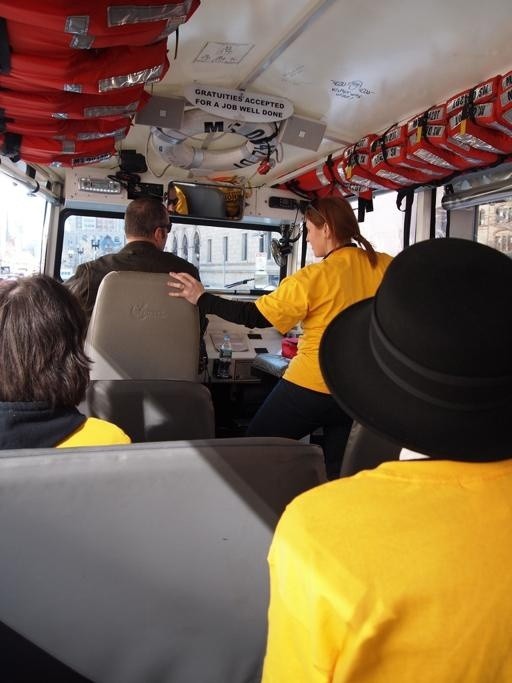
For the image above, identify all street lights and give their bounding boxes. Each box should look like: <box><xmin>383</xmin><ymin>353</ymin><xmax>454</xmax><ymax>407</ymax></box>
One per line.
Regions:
<box><xmin>62</xmin><ymin>239</ymin><xmax>100</xmax><ymax>273</ymax></box>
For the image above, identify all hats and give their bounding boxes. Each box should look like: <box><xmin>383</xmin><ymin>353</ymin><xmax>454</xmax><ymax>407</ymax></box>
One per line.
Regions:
<box><xmin>319</xmin><ymin>238</ymin><xmax>512</xmax><ymax>461</ymax></box>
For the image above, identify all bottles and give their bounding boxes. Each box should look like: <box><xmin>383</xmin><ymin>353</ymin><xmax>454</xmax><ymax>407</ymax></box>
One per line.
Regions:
<box><xmin>218</xmin><ymin>335</ymin><xmax>233</xmax><ymax>378</ymax></box>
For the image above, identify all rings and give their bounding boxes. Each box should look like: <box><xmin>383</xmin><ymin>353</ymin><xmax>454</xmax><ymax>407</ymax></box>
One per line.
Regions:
<box><xmin>180</xmin><ymin>284</ymin><xmax>185</xmax><ymax>291</ymax></box>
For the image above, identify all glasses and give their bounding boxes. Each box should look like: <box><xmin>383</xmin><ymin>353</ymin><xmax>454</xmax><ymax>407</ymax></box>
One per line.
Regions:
<box><xmin>161</xmin><ymin>222</ymin><xmax>172</xmax><ymax>232</ymax></box>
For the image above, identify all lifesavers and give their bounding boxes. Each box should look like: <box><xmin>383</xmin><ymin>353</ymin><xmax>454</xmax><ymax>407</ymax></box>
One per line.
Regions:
<box><xmin>153</xmin><ymin>108</ymin><xmax>280</xmax><ymax>169</ymax></box>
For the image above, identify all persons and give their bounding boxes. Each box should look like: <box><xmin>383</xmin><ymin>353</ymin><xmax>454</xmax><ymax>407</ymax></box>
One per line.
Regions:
<box><xmin>262</xmin><ymin>236</ymin><xmax>512</xmax><ymax>683</ymax></box>
<box><xmin>0</xmin><ymin>274</ymin><xmax>132</xmax><ymax>451</ymax></box>
<box><xmin>166</xmin><ymin>196</ymin><xmax>396</xmax><ymax>478</ymax></box>
<box><xmin>59</xmin><ymin>197</ymin><xmax>210</xmax><ymax>373</ymax></box>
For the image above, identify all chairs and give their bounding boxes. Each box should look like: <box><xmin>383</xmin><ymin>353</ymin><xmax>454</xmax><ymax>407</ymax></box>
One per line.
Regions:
<box><xmin>339</xmin><ymin>418</ymin><xmax>400</xmax><ymax>478</ymax></box>
<box><xmin>77</xmin><ymin>379</ymin><xmax>215</xmax><ymax>443</ymax></box>
<box><xmin>82</xmin><ymin>271</ymin><xmax>201</xmax><ymax>380</ymax></box>
<box><xmin>0</xmin><ymin>436</ymin><xmax>327</xmax><ymax>683</ymax></box>
